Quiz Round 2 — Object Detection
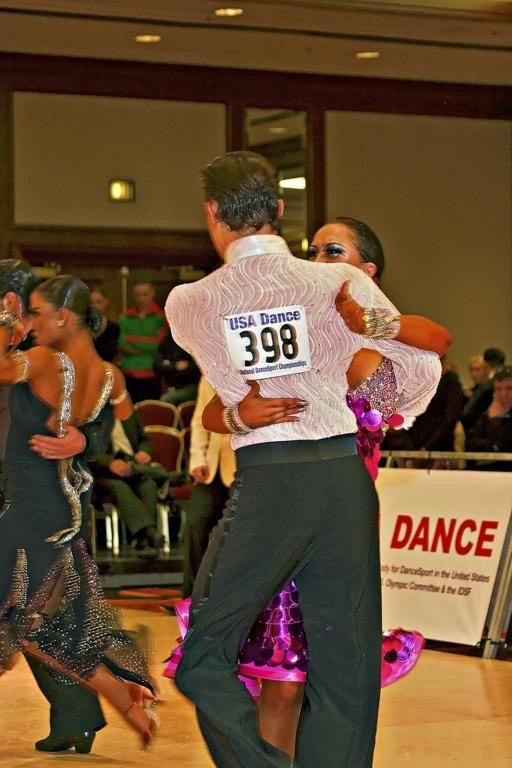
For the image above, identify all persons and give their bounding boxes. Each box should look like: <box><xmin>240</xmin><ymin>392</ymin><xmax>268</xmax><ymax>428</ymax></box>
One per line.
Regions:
<box><xmin>1</xmin><ymin>257</ymin><xmax>103</xmax><ymax>755</ymax></box>
<box><xmin>381</xmin><ymin>345</ymin><xmax>512</xmax><ymax>470</ymax></box>
<box><xmin>79</xmin><ymin>282</ymin><xmax>199</xmax><ymax>402</ymax></box>
<box><xmin>1</xmin><ymin>276</ymin><xmax>161</xmax><ymax>751</ymax></box>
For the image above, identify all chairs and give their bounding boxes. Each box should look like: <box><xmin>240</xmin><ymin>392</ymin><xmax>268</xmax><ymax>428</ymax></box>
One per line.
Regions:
<box><xmin>89</xmin><ymin>399</ymin><xmax>197</xmax><ymax>556</ymax></box>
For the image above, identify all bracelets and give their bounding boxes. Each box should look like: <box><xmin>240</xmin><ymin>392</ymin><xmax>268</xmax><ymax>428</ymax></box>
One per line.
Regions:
<box><xmin>220</xmin><ymin>404</ymin><xmax>254</xmax><ymax>440</ymax></box>
<box><xmin>363</xmin><ymin>304</ymin><xmax>404</xmax><ymax>341</ymax></box>
<box><xmin>1</xmin><ymin>313</ymin><xmax>19</xmax><ymax>335</ymax></box>
